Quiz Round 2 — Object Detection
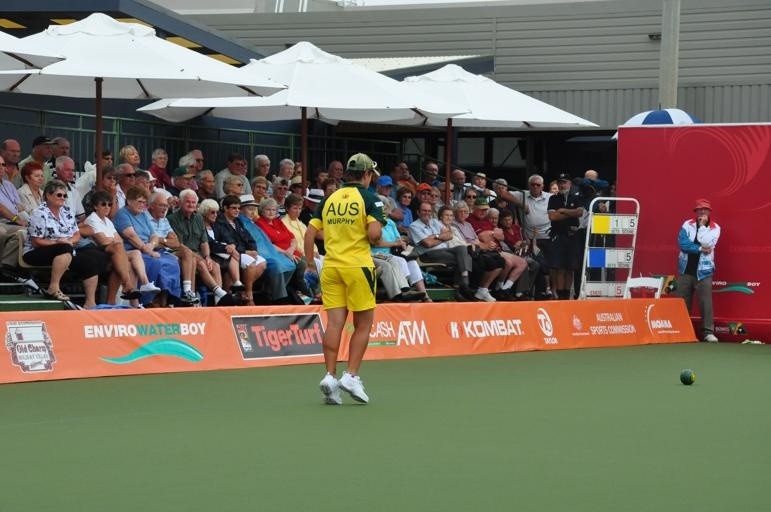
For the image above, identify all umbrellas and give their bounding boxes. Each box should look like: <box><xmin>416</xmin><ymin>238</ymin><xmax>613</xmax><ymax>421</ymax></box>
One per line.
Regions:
<box><xmin>608</xmin><ymin>103</ymin><xmax>705</xmax><ymax>146</ymax></box>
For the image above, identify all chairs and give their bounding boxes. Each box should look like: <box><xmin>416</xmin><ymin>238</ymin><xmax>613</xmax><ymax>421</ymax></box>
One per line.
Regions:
<box><xmin>621</xmin><ymin>277</ymin><xmax>665</xmax><ymax>298</ymax></box>
<box><xmin>16</xmin><ymin>228</ymin><xmax>69</xmax><ymax>269</ymax></box>
<box><xmin>417</xmin><ymin>254</ymin><xmax>457</xmax><ymax>269</ymax></box>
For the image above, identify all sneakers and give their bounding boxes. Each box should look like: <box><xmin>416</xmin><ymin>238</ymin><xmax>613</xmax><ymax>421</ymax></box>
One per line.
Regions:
<box><xmin>140</xmin><ymin>281</ymin><xmax>162</xmax><ymax>296</ymax></box>
<box><xmin>475</xmin><ymin>287</ymin><xmax>496</xmax><ymax>302</ymax></box>
<box><xmin>338</xmin><ymin>371</ymin><xmax>369</xmax><ymax>403</ymax></box>
<box><xmin>319</xmin><ymin>374</ymin><xmax>342</xmax><ymax>405</ymax></box>
<box><xmin>704</xmin><ymin>334</ymin><xmax>717</xmax><ymax>342</ymax></box>
<box><xmin>180</xmin><ymin>291</ymin><xmax>200</xmax><ymax>303</ymax></box>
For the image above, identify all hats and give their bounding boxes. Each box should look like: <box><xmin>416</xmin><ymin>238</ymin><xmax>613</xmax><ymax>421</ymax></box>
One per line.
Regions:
<box><xmin>304</xmin><ymin>188</ymin><xmax>325</xmax><ymax>203</ymax></box>
<box><xmin>347</xmin><ymin>152</ymin><xmax>380</xmax><ymax>177</ymax></box>
<box><xmin>557</xmin><ymin>173</ymin><xmax>572</xmax><ymax>182</ymax></box>
<box><xmin>32</xmin><ymin>135</ymin><xmax>60</xmax><ymax>147</ymax></box>
<box><xmin>175</xmin><ymin>166</ymin><xmax>197</xmax><ymax>178</ymax></box>
<box><xmin>471</xmin><ymin>197</ymin><xmax>490</xmax><ymax>210</ymax></box>
<box><xmin>238</xmin><ymin>194</ymin><xmax>260</xmax><ymax>206</ymax></box>
<box><xmin>375</xmin><ymin>175</ymin><xmax>393</xmax><ymax>187</ymax></box>
<box><xmin>416</xmin><ymin>183</ymin><xmax>432</xmax><ymax>192</ymax></box>
<box><xmin>693</xmin><ymin>198</ymin><xmax>712</xmax><ymax>212</ymax></box>
<box><xmin>288</xmin><ymin>176</ymin><xmax>311</xmax><ymax>191</ymax></box>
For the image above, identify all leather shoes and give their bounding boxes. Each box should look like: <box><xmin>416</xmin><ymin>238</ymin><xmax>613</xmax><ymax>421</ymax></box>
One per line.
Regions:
<box><xmin>402</xmin><ymin>290</ymin><xmax>425</xmax><ymax>300</ymax></box>
<box><xmin>230</xmin><ymin>284</ymin><xmax>248</xmax><ymax>291</ymax></box>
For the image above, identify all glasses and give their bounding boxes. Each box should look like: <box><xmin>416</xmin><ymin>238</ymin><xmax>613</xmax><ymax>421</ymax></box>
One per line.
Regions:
<box><xmin>422</xmin><ymin>191</ymin><xmax>430</xmax><ymax>196</ymax></box>
<box><xmin>238</xmin><ymin>184</ymin><xmax>244</xmax><ymax>186</ymax></box>
<box><xmin>233</xmin><ymin>207</ymin><xmax>241</xmax><ymax>209</ymax></box>
<box><xmin>467</xmin><ymin>195</ymin><xmax>477</xmax><ymax>199</ymax></box>
<box><xmin>451</xmin><ymin>189</ymin><xmax>454</xmax><ymax>193</ymax></box>
<box><xmin>531</xmin><ymin>183</ymin><xmax>540</xmax><ymax>187</ymax></box>
<box><xmin>0</xmin><ymin>158</ymin><xmax>136</xmax><ymax>207</ymax></box>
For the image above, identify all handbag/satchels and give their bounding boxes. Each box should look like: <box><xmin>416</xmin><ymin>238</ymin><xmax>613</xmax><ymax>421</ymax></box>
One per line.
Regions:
<box><xmin>392</xmin><ymin>241</ymin><xmax>418</xmax><ymax>261</ymax></box>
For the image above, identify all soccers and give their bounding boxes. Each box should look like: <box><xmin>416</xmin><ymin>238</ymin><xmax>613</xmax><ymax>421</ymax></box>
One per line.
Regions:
<box><xmin>680</xmin><ymin>369</ymin><xmax>697</xmax><ymax>386</ymax></box>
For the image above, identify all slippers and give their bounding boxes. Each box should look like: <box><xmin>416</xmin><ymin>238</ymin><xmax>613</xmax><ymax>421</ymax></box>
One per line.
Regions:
<box><xmin>44</xmin><ymin>290</ymin><xmax>71</xmax><ymax>301</ymax></box>
<box><xmin>121</xmin><ymin>288</ymin><xmax>142</xmax><ymax>299</ymax></box>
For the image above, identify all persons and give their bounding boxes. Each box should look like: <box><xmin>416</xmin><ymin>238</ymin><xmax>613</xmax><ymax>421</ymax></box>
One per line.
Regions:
<box><xmin>1</xmin><ymin>136</ymin><xmax>616</xmax><ymax>310</ymax></box>
<box><xmin>676</xmin><ymin>197</ymin><xmax>723</xmax><ymax>344</ymax></box>
<box><xmin>304</xmin><ymin>150</ymin><xmax>387</xmax><ymax>408</ymax></box>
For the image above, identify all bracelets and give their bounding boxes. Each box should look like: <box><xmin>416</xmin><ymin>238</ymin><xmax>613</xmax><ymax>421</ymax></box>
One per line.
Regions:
<box><xmin>307</xmin><ymin>261</ymin><xmax>316</xmax><ymax>266</ymax></box>
<box><xmin>698</xmin><ymin>246</ymin><xmax>704</xmax><ymax>250</ymax></box>
